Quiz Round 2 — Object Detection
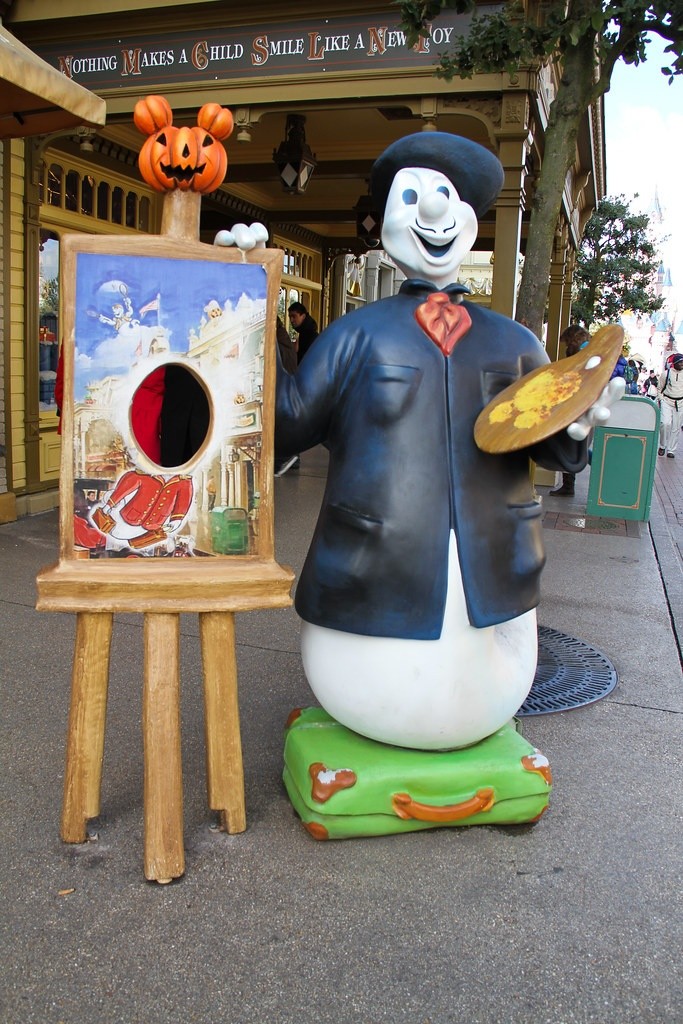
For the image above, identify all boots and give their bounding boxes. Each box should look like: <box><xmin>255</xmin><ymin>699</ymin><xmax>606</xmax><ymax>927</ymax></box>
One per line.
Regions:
<box><xmin>550</xmin><ymin>472</ymin><xmax>575</xmax><ymax>496</ymax></box>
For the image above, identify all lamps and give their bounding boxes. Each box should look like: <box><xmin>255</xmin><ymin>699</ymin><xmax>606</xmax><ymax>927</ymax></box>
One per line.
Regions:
<box><xmin>352</xmin><ymin>178</ymin><xmax>383</xmax><ymax>248</ymax></box>
<box><xmin>271</xmin><ymin>114</ymin><xmax>318</xmax><ymax>197</ymax></box>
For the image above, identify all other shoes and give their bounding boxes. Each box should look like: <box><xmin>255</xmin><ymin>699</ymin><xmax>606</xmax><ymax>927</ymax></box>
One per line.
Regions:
<box><xmin>275</xmin><ymin>453</ymin><xmax>300</xmax><ymax>478</ymax></box>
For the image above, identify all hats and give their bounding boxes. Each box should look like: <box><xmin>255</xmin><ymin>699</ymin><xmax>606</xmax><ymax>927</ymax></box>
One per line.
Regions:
<box><xmin>672</xmin><ymin>353</ymin><xmax>683</xmax><ymax>364</ymax></box>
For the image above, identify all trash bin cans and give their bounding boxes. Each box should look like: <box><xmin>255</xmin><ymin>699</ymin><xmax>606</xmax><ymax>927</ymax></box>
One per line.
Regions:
<box><xmin>585</xmin><ymin>395</ymin><xmax>661</xmax><ymax>523</ymax></box>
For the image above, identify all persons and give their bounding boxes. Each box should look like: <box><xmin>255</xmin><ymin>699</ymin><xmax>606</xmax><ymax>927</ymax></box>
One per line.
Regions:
<box><xmin>288</xmin><ymin>302</ymin><xmax>319</xmax><ymax>468</ymax></box>
<box><xmin>612</xmin><ymin>354</ymin><xmax>658</xmax><ymax>400</ymax></box>
<box><xmin>274</xmin><ymin>317</ymin><xmax>298</xmax><ymax>477</ymax></box>
<box><xmin>550</xmin><ymin>324</ymin><xmax>592</xmax><ymax>501</ymax></box>
<box><xmin>658</xmin><ymin>353</ymin><xmax>683</xmax><ymax>458</ymax></box>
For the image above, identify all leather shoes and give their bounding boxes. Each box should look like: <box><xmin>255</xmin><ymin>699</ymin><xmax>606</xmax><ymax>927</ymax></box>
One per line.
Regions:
<box><xmin>658</xmin><ymin>448</ymin><xmax>665</xmax><ymax>456</ymax></box>
<box><xmin>667</xmin><ymin>453</ymin><xmax>675</xmax><ymax>458</ymax></box>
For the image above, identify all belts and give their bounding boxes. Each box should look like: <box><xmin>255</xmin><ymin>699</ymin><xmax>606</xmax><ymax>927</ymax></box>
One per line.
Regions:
<box><xmin>663</xmin><ymin>393</ymin><xmax>683</xmax><ymax>400</ymax></box>
